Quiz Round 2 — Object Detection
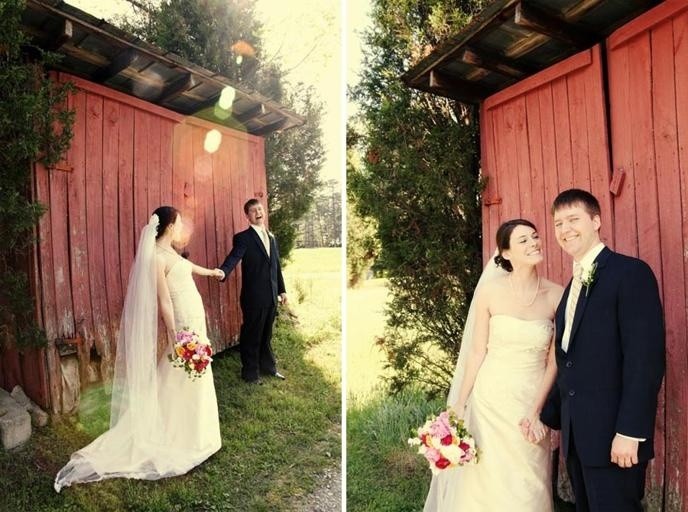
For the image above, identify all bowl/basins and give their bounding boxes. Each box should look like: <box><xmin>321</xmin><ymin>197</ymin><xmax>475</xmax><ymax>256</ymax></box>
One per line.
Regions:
<box><xmin>156</xmin><ymin>243</ymin><xmax>180</xmax><ymax>258</ymax></box>
<box><xmin>508</xmin><ymin>268</ymin><xmax>541</xmax><ymax>307</ymax></box>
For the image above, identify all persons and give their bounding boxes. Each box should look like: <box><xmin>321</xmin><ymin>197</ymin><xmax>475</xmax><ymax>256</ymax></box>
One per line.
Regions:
<box><xmin>528</xmin><ymin>189</ymin><xmax>667</xmax><ymax>511</ymax></box>
<box><xmin>214</xmin><ymin>196</ymin><xmax>288</xmax><ymax>384</ymax></box>
<box><xmin>448</xmin><ymin>220</ymin><xmax>571</xmax><ymax>511</ymax></box>
<box><xmin>136</xmin><ymin>206</ymin><xmax>224</xmax><ymax>469</ymax></box>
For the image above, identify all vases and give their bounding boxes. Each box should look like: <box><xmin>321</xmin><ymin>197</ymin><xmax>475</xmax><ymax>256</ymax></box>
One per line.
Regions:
<box><xmin>578</xmin><ymin>260</ymin><xmax>597</xmax><ymax>286</ymax></box>
<box><xmin>168</xmin><ymin>327</ymin><xmax>214</xmax><ymax>377</ymax></box>
<box><xmin>408</xmin><ymin>409</ymin><xmax>481</xmax><ymax>475</ymax></box>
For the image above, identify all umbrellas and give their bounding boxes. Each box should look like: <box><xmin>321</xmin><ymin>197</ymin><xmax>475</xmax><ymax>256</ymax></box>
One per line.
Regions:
<box><xmin>271</xmin><ymin>372</ymin><xmax>285</xmax><ymax>382</ymax></box>
<box><xmin>248</xmin><ymin>379</ymin><xmax>263</xmax><ymax>385</ymax></box>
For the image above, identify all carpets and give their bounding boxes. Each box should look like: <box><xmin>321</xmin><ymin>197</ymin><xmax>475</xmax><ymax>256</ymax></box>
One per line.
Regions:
<box><xmin>561</xmin><ymin>263</ymin><xmax>583</xmax><ymax>352</ymax></box>
<box><xmin>262</xmin><ymin>229</ymin><xmax>270</xmax><ymax>258</ymax></box>
